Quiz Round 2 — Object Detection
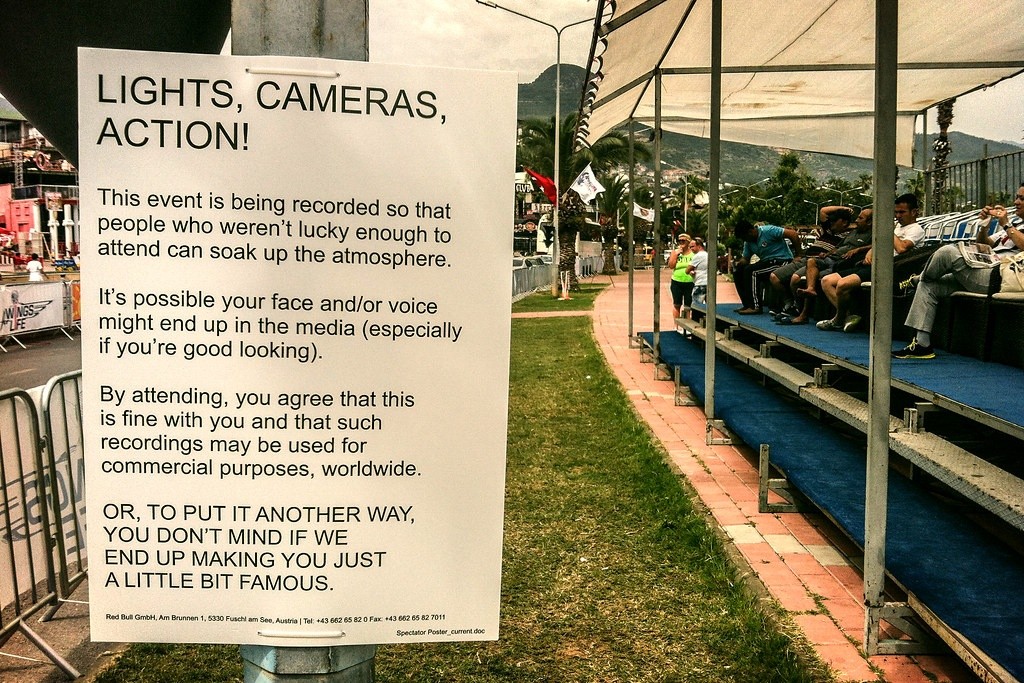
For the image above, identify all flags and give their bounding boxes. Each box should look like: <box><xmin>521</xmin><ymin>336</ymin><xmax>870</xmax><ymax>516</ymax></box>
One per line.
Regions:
<box><xmin>571</xmin><ymin>166</ymin><xmax>606</xmax><ymax>205</ymax></box>
<box><xmin>525</xmin><ymin>169</ymin><xmax>555</xmax><ymax>202</ymax></box>
<box><xmin>632</xmin><ymin>202</ymin><xmax>655</xmax><ymax>222</ymax></box>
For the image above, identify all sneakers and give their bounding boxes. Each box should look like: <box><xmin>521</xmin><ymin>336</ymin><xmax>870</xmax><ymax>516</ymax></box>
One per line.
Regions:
<box><xmin>893</xmin><ymin>273</ymin><xmax>916</xmax><ymax>297</ymax></box>
<box><xmin>891</xmin><ymin>337</ymin><xmax>936</xmax><ymax>359</ymax></box>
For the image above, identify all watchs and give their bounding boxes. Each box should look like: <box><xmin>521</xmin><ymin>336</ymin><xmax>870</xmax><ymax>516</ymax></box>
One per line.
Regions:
<box><xmin>1004</xmin><ymin>222</ymin><xmax>1013</xmax><ymax>232</ymax></box>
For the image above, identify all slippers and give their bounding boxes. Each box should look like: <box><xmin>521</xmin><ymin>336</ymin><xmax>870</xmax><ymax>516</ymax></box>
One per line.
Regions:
<box><xmin>770</xmin><ymin>314</ymin><xmax>805</xmax><ymax>325</ymax></box>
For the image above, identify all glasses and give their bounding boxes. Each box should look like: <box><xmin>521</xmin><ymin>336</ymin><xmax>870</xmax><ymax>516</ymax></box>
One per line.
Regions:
<box><xmin>678</xmin><ymin>239</ymin><xmax>686</xmax><ymax>242</ymax></box>
<box><xmin>692</xmin><ymin>238</ymin><xmax>697</xmax><ymax>244</ymax></box>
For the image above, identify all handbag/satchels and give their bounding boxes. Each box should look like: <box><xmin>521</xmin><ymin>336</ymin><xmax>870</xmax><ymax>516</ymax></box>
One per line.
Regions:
<box><xmin>999</xmin><ymin>251</ymin><xmax>1024</xmax><ymax>292</ymax></box>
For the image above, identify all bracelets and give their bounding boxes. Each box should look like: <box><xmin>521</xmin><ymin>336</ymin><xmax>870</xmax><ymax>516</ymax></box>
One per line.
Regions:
<box><xmin>1008</xmin><ymin>229</ymin><xmax>1016</xmax><ymax>238</ymax></box>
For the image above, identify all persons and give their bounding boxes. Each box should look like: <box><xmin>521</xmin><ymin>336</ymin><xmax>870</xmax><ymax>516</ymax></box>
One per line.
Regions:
<box><xmin>553</xmin><ymin>192</ymin><xmax>709</xmax><ymax>337</ymax></box>
<box><xmin>730</xmin><ymin>192</ymin><xmax>928</xmax><ymax>331</ymax></box>
<box><xmin>0</xmin><ymin>160</ymin><xmax>71</xmax><ymax>284</ymax></box>
<box><xmin>891</xmin><ymin>183</ymin><xmax>1024</xmax><ymax>360</ymax></box>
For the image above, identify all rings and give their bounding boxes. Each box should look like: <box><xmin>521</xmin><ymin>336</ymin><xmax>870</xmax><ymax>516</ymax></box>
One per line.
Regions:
<box><xmin>997</xmin><ymin>212</ymin><xmax>1001</xmax><ymax>214</ymax></box>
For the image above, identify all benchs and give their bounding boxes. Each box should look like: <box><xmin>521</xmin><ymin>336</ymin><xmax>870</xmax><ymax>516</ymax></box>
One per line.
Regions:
<box><xmin>632</xmin><ymin>279</ymin><xmax>1024</xmax><ymax>683</ymax></box>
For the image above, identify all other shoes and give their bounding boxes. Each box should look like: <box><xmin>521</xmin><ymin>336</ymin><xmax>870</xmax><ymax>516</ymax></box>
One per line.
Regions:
<box><xmin>844</xmin><ymin>313</ymin><xmax>862</xmax><ymax>332</ymax></box>
<box><xmin>733</xmin><ymin>306</ymin><xmax>763</xmax><ymax>315</ymax></box>
<box><xmin>769</xmin><ymin>305</ymin><xmax>801</xmax><ymax>316</ymax></box>
<box><xmin>816</xmin><ymin>319</ymin><xmax>844</xmax><ymax>331</ymax></box>
